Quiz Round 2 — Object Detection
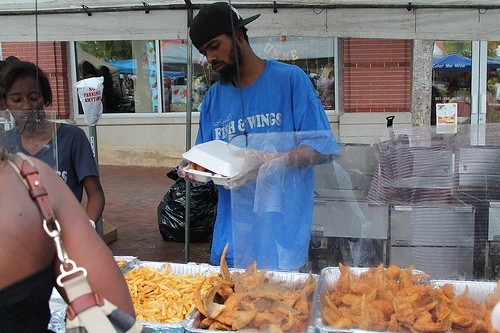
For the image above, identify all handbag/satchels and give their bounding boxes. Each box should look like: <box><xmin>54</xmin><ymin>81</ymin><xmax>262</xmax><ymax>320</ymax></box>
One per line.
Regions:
<box><xmin>8</xmin><ymin>152</ymin><xmax>152</xmax><ymax>333</ymax></box>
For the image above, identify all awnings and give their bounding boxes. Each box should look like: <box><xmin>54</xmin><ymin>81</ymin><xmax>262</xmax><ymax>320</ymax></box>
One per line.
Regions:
<box><xmin>431</xmin><ymin>52</ymin><xmax>500</xmax><ymax>70</ymax></box>
<box><xmin>106</xmin><ymin>37</ymin><xmax>336</xmax><ymax>75</ymax></box>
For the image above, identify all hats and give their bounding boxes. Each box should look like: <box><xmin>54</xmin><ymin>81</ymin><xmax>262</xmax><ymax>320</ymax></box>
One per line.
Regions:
<box><xmin>190</xmin><ymin>2</ymin><xmax>261</xmax><ymax>50</ymax></box>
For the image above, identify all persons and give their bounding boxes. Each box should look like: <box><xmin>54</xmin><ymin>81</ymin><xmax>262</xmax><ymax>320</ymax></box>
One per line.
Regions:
<box><xmin>1</xmin><ymin>55</ymin><xmax>106</xmax><ymax>257</ymax></box>
<box><xmin>176</xmin><ymin>2</ymin><xmax>343</xmax><ymax>272</ymax></box>
<box><xmin>0</xmin><ymin>151</ymin><xmax>136</xmax><ymax>333</ymax></box>
<box><xmin>352</xmin><ymin>85</ymin><xmax>453</xmax><ymax>277</ymax></box>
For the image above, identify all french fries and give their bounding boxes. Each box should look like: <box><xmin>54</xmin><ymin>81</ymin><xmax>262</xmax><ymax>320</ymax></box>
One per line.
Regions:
<box><xmin>124</xmin><ymin>263</ymin><xmax>213</xmax><ymax>324</ymax></box>
